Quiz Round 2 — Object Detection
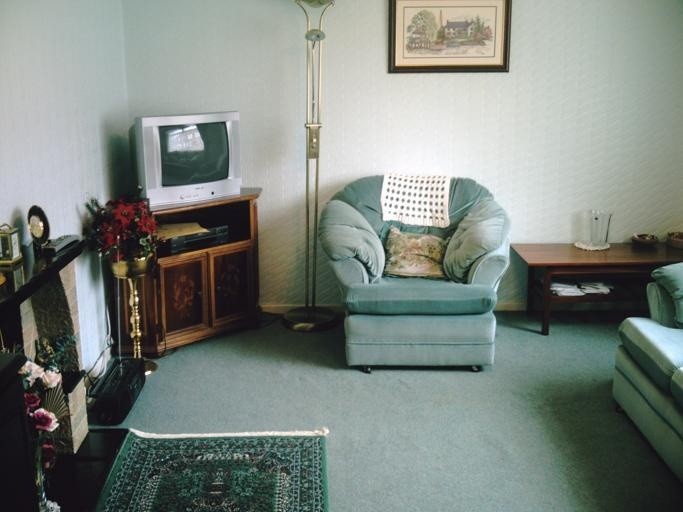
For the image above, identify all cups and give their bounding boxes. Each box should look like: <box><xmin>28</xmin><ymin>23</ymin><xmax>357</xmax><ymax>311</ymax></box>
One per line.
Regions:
<box><xmin>591</xmin><ymin>209</ymin><xmax>613</xmax><ymax>247</ymax></box>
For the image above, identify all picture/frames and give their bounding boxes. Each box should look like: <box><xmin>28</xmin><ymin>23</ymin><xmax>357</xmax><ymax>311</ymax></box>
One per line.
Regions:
<box><xmin>388</xmin><ymin>0</ymin><xmax>512</xmax><ymax>72</ymax></box>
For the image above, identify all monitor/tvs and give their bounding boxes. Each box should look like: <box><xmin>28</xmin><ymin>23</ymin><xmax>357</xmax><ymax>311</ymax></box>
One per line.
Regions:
<box><xmin>129</xmin><ymin>110</ymin><xmax>241</xmax><ymax>212</ymax></box>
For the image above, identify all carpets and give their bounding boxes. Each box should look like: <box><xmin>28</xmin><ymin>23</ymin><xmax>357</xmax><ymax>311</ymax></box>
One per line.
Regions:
<box><xmin>92</xmin><ymin>428</ymin><xmax>328</xmax><ymax>512</ymax></box>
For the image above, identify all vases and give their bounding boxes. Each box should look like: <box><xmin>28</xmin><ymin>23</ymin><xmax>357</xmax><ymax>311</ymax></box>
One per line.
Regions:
<box><xmin>106</xmin><ymin>252</ymin><xmax>153</xmax><ymax>277</ymax></box>
<box><xmin>38</xmin><ymin>492</ymin><xmax>60</xmax><ymax>511</ymax></box>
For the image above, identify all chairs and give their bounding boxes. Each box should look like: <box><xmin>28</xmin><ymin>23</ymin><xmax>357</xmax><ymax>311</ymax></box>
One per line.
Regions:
<box><xmin>317</xmin><ymin>175</ymin><xmax>512</xmax><ymax>372</ymax></box>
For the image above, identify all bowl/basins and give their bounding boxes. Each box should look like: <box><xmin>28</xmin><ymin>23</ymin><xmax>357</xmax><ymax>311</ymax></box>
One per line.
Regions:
<box><xmin>667</xmin><ymin>231</ymin><xmax>683</xmax><ymax>250</ymax></box>
<box><xmin>632</xmin><ymin>232</ymin><xmax>659</xmax><ymax>246</ymax></box>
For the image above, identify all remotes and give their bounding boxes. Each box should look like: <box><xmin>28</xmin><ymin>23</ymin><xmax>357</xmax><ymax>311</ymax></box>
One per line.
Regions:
<box><xmin>45</xmin><ymin>233</ymin><xmax>79</xmax><ymax>254</ymax></box>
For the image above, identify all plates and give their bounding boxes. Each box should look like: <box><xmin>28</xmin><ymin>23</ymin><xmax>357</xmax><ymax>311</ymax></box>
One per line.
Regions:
<box><xmin>574</xmin><ymin>240</ymin><xmax>611</xmax><ymax>251</ymax></box>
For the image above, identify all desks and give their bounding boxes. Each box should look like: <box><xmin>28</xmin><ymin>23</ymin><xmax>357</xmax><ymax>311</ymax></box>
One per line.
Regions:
<box><xmin>507</xmin><ymin>241</ymin><xmax>683</xmax><ymax>335</ymax></box>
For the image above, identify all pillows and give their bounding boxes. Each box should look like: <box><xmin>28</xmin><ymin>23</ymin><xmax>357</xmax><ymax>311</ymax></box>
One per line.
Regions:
<box><xmin>383</xmin><ymin>224</ymin><xmax>451</xmax><ymax>280</ymax></box>
<box><xmin>650</xmin><ymin>262</ymin><xmax>682</xmax><ymax>329</ymax></box>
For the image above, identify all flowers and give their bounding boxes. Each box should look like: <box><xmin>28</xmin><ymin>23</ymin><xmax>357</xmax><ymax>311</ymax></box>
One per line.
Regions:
<box><xmin>81</xmin><ymin>191</ymin><xmax>158</xmax><ymax>263</ymax></box>
<box><xmin>18</xmin><ymin>359</ymin><xmax>72</xmax><ymax>496</ymax></box>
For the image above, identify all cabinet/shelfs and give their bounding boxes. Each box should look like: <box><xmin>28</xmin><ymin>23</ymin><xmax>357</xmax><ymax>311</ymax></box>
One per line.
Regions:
<box><xmin>112</xmin><ymin>187</ymin><xmax>263</xmax><ymax>357</ymax></box>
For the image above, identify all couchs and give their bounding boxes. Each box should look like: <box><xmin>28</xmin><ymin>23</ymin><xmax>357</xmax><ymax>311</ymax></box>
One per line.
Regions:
<box><xmin>611</xmin><ymin>282</ymin><xmax>682</xmax><ymax>486</ymax></box>
